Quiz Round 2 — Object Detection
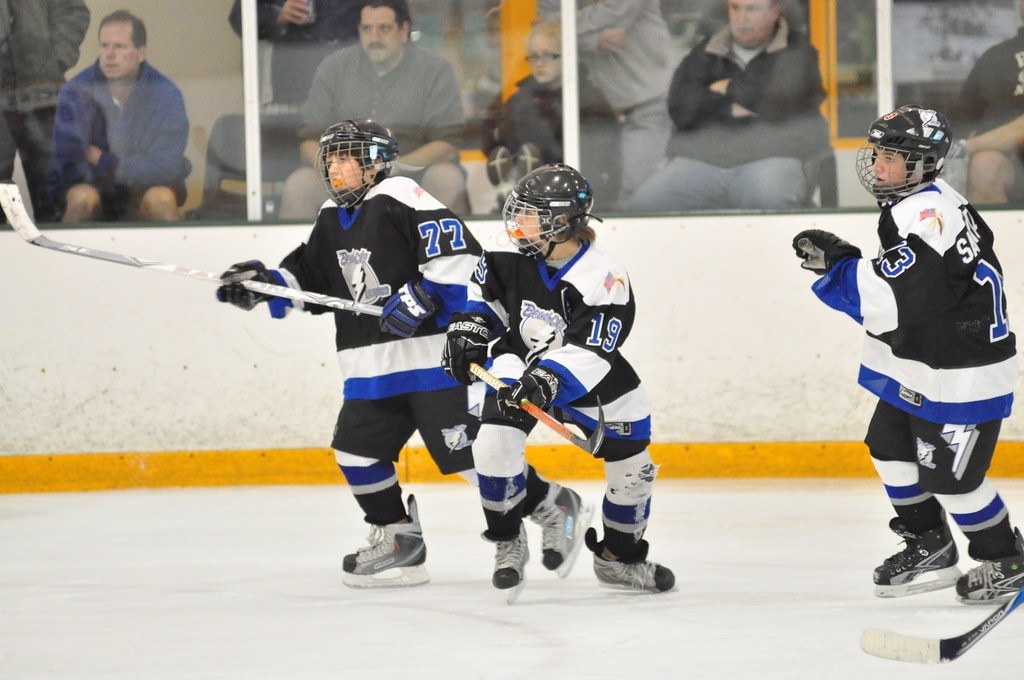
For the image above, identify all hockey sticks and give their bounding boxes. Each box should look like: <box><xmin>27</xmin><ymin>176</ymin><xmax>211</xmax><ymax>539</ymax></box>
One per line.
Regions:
<box><xmin>468</xmin><ymin>360</ymin><xmax>607</xmax><ymax>456</ymax></box>
<box><xmin>797</xmin><ymin>236</ymin><xmax>825</xmax><ymax>259</ymax></box>
<box><xmin>0</xmin><ymin>178</ymin><xmax>385</xmax><ymax>317</ymax></box>
<box><xmin>860</xmin><ymin>584</ymin><xmax>1024</xmax><ymax>666</ymax></box>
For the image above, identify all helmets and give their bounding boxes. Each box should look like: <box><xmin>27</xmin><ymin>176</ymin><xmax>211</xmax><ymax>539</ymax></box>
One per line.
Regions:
<box><xmin>855</xmin><ymin>105</ymin><xmax>953</xmax><ymax>210</ymax></box>
<box><xmin>315</xmin><ymin>117</ymin><xmax>398</xmax><ymax>210</ymax></box>
<box><xmin>503</xmin><ymin>163</ymin><xmax>594</xmax><ymax>260</ymax></box>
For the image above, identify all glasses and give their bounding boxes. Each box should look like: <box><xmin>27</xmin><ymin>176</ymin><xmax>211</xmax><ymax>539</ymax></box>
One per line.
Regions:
<box><xmin>524</xmin><ymin>51</ymin><xmax>560</xmax><ymax>64</ymax></box>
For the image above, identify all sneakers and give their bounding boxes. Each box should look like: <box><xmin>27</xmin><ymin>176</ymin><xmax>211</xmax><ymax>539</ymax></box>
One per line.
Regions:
<box><xmin>956</xmin><ymin>529</ymin><xmax>1024</xmax><ymax>605</ymax></box>
<box><xmin>342</xmin><ymin>495</ymin><xmax>431</xmax><ymax>588</ymax></box>
<box><xmin>480</xmin><ymin>524</ymin><xmax>529</xmax><ymax>604</ymax></box>
<box><xmin>522</xmin><ymin>475</ymin><xmax>596</xmax><ymax>577</ymax></box>
<box><xmin>584</xmin><ymin>527</ymin><xmax>675</xmax><ymax>593</ymax></box>
<box><xmin>873</xmin><ymin>507</ymin><xmax>968</xmax><ymax>598</ymax></box>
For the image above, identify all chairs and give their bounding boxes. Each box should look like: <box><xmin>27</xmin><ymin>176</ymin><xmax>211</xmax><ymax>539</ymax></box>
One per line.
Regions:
<box><xmin>222</xmin><ymin>95</ymin><xmax>499</xmax><ymax>214</ymax></box>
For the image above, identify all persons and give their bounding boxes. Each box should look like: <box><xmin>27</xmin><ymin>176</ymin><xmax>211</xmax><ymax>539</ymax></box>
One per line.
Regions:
<box><xmin>442</xmin><ymin>163</ymin><xmax>675</xmax><ymax>592</ymax></box>
<box><xmin>216</xmin><ymin>116</ymin><xmax>593</xmax><ymax>587</ymax></box>
<box><xmin>0</xmin><ymin>0</ymin><xmax>1024</xmax><ymax>221</ymax></box>
<box><xmin>793</xmin><ymin>104</ymin><xmax>1024</xmax><ymax>605</ymax></box>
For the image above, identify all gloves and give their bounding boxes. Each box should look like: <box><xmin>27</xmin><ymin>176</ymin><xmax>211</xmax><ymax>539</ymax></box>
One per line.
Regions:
<box><xmin>218</xmin><ymin>261</ymin><xmax>274</xmax><ymax>310</ymax></box>
<box><xmin>792</xmin><ymin>230</ymin><xmax>861</xmax><ymax>275</ymax></box>
<box><xmin>441</xmin><ymin>310</ymin><xmax>492</xmax><ymax>386</ymax></box>
<box><xmin>497</xmin><ymin>368</ymin><xmax>561</xmax><ymax>419</ymax></box>
<box><xmin>378</xmin><ymin>284</ymin><xmax>436</xmax><ymax>338</ymax></box>
